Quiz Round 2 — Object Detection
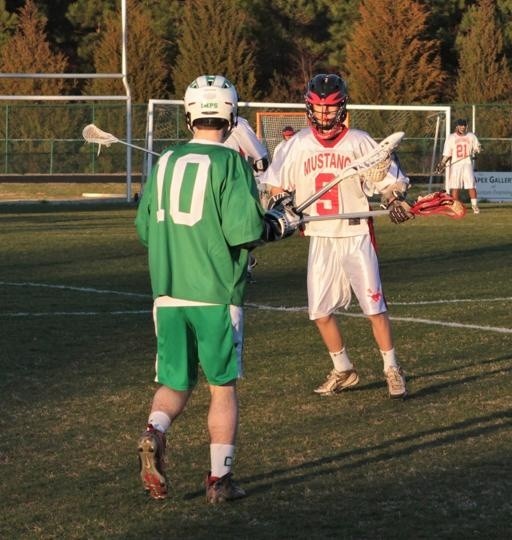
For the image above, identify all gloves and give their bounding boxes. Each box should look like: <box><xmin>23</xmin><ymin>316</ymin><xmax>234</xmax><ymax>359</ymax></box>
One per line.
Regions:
<box><xmin>263</xmin><ymin>202</ymin><xmax>304</xmax><ymax>241</ymax></box>
<box><xmin>432</xmin><ymin>161</ymin><xmax>446</xmax><ymax>175</ymax></box>
<box><xmin>267</xmin><ymin>191</ymin><xmax>295</xmax><ymax>213</ymax></box>
<box><xmin>379</xmin><ymin>190</ymin><xmax>415</xmax><ymax>224</ymax></box>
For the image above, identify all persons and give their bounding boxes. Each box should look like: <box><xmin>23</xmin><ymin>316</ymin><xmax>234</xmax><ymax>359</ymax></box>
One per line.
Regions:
<box><xmin>271</xmin><ymin>124</ymin><xmax>295</xmax><ymax>163</ymax></box>
<box><xmin>133</xmin><ymin>75</ymin><xmax>299</xmax><ymax>502</ymax></box>
<box><xmin>433</xmin><ymin>119</ymin><xmax>482</xmax><ymax>215</ymax></box>
<box><xmin>222</xmin><ymin>116</ymin><xmax>270</xmax><ymax>286</ymax></box>
<box><xmin>259</xmin><ymin>73</ymin><xmax>414</xmax><ymax>400</ymax></box>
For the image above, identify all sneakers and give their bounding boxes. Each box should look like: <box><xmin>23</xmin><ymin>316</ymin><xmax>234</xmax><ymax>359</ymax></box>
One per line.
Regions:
<box><xmin>313</xmin><ymin>366</ymin><xmax>359</xmax><ymax>396</ymax></box>
<box><xmin>472</xmin><ymin>204</ymin><xmax>480</xmax><ymax>214</ymax></box>
<box><xmin>204</xmin><ymin>470</ymin><xmax>247</xmax><ymax>503</ymax></box>
<box><xmin>137</xmin><ymin>422</ymin><xmax>170</xmax><ymax>500</ymax></box>
<box><xmin>383</xmin><ymin>366</ymin><xmax>407</xmax><ymax>397</ymax></box>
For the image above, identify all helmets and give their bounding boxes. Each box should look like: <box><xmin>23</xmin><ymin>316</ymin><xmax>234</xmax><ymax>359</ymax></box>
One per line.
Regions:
<box><xmin>454</xmin><ymin>119</ymin><xmax>467</xmax><ymax>127</ymax></box>
<box><xmin>282</xmin><ymin>126</ymin><xmax>295</xmax><ymax>141</ymax></box>
<box><xmin>304</xmin><ymin>73</ymin><xmax>348</xmax><ymax>123</ymax></box>
<box><xmin>183</xmin><ymin>74</ymin><xmax>238</xmax><ymax>136</ymax></box>
